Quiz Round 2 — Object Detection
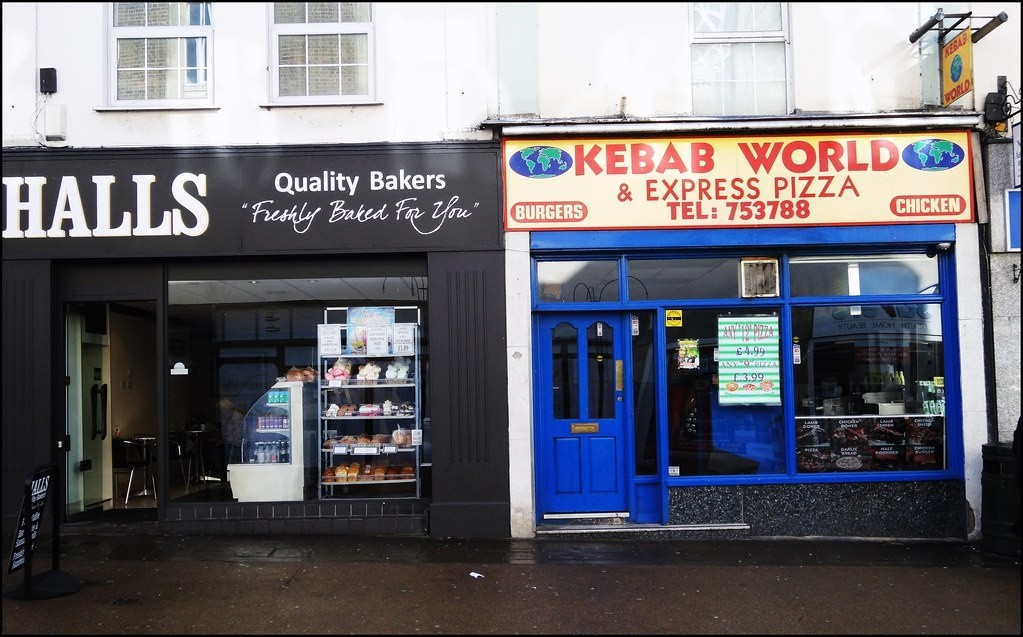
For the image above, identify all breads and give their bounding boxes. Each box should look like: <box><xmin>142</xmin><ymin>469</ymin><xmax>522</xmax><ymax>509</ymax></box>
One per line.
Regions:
<box><xmin>321</xmin><ymin>358</ymin><xmax>417</xmax><ymax>482</ymax></box>
<box><xmin>287</xmin><ymin>368</ymin><xmax>318</xmax><ymax>383</ymax></box>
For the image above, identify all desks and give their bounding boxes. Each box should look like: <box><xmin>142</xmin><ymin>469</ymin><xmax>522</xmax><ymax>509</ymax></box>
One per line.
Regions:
<box><xmin>134</xmin><ymin>437</ymin><xmax>155</xmax><ymax>496</ymax></box>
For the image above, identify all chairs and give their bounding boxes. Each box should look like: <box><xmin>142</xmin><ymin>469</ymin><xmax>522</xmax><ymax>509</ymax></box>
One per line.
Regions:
<box><xmin>112</xmin><ymin>432</ymin><xmax>206</xmax><ymax>506</ymax></box>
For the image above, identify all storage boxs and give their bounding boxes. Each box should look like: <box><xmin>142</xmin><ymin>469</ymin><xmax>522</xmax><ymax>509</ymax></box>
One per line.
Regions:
<box><xmin>863</xmin><ymin>402</ymin><xmax>905</xmax><ymax>415</ymax></box>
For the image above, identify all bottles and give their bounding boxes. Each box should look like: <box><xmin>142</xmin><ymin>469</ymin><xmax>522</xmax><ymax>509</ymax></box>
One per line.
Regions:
<box><xmin>250</xmin><ymin>441</ymin><xmax>290</xmax><ymax>463</ymax></box>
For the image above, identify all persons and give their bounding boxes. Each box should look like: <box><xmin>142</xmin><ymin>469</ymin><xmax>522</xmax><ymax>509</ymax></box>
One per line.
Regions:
<box><xmin>215</xmin><ymin>399</ymin><xmax>249</xmax><ymax>464</ymax></box>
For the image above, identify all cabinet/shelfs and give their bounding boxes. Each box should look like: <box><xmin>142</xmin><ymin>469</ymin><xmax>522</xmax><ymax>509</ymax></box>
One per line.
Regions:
<box><xmin>227</xmin><ymin>381</ymin><xmax>317</xmax><ymax>503</ymax></box>
<box><xmin>318</xmin><ymin>306</ymin><xmax>420</xmax><ymax>499</ymax></box>
